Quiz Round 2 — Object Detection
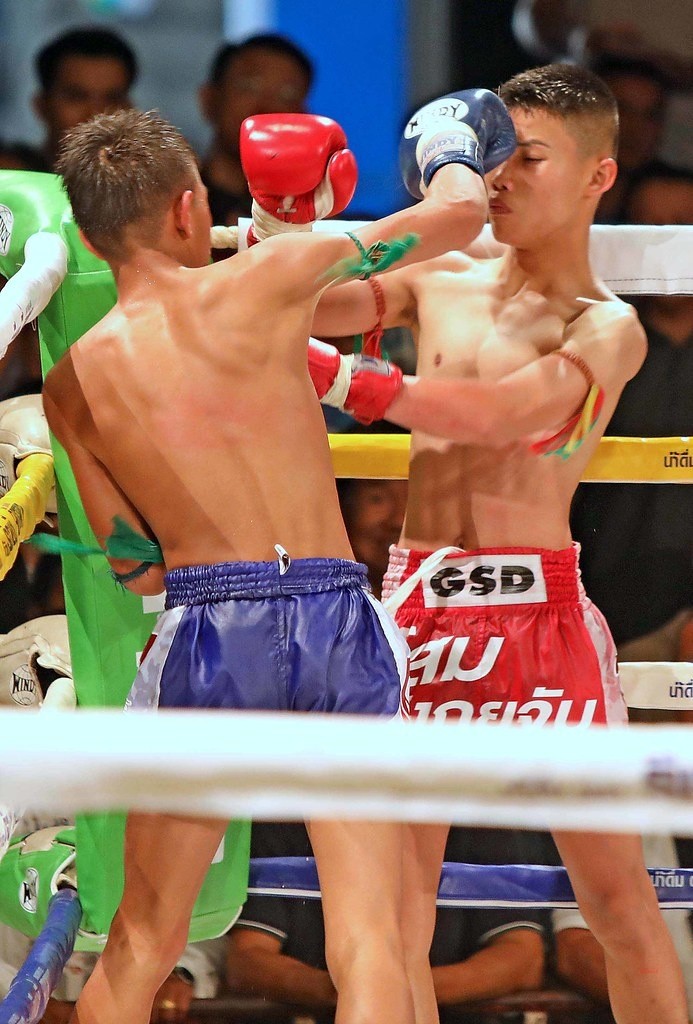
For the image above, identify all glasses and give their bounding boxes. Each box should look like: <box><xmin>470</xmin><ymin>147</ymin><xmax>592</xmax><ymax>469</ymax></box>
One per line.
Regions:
<box><xmin>52</xmin><ymin>85</ymin><xmax>127</xmax><ymax>105</ymax></box>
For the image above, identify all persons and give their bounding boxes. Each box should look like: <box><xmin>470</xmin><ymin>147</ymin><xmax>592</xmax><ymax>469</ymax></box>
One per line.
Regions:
<box><xmin>0</xmin><ymin>23</ymin><xmax>693</xmax><ymax>1024</ymax></box>
<box><xmin>241</xmin><ymin>66</ymin><xmax>688</xmax><ymax>1024</ymax></box>
<box><xmin>43</xmin><ymin>88</ymin><xmax>517</xmax><ymax>1024</ymax></box>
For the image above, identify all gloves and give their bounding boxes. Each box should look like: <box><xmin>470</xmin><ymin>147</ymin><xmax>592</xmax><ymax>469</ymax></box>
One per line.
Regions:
<box><xmin>394</xmin><ymin>88</ymin><xmax>518</xmax><ymax>198</ymax></box>
<box><xmin>306</xmin><ymin>337</ymin><xmax>402</xmax><ymax>426</ymax></box>
<box><xmin>239</xmin><ymin>113</ymin><xmax>358</xmax><ymax>244</ymax></box>
